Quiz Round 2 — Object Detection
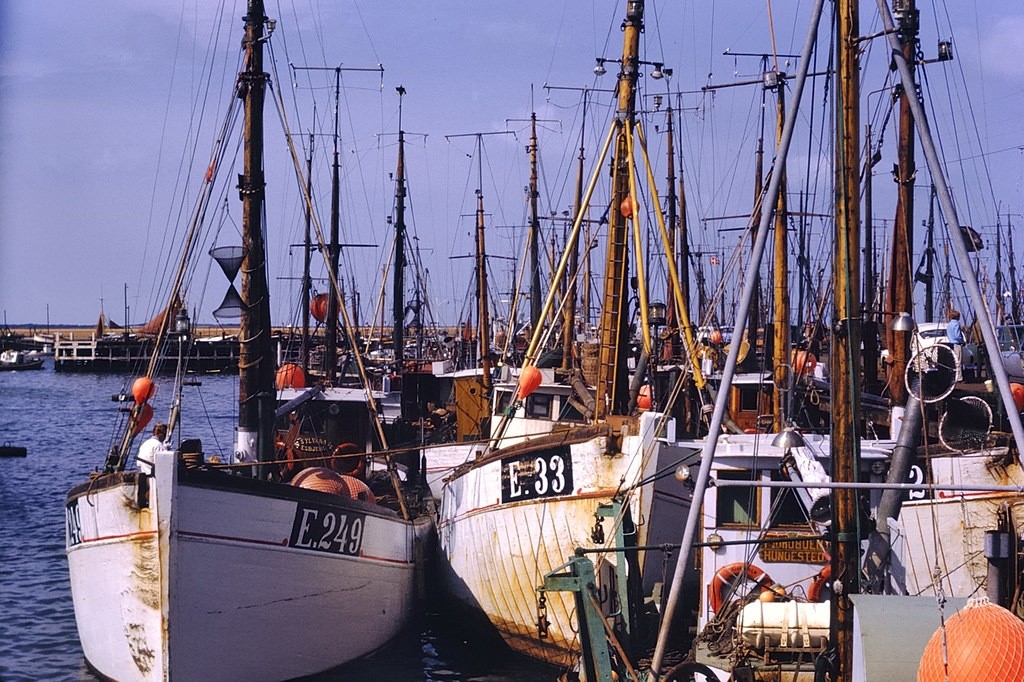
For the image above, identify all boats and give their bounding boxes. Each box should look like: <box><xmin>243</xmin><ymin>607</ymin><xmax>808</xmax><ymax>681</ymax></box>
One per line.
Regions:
<box><xmin>0</xmin><ymin>347</ymin><xmax>47</xmax><ymax>370</ymax></box>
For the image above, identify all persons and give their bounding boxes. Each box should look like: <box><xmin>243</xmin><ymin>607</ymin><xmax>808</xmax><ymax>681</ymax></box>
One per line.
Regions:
<box><xmin>137</xmin><ymin>422</ymin><xmax>170</xmax><ymax>477</ymax></box>
<box><xmin>947</xmin><ymin>311</ymin><xmax>967</xmax><ymax>383</ymax></box>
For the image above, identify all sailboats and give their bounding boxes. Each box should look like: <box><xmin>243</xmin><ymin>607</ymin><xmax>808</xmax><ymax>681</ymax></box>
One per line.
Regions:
<box><xmin>63</xmin><ymin>0</ymin><xmax>430</xmax><ymax>682</ymax></box>
<box><xmin>33</xmin><ymin>303</ymin><xmax>65</xmax><ymax>344</ymax></box>
<box><xmin>418</xmin><ymin>0</ymin><xmax>1024</xmax><ymax>682</ymax></box>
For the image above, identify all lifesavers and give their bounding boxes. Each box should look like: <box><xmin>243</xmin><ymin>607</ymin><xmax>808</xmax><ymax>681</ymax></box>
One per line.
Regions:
<box><xmin>276</xmin><ymin>441</ymin><xmax>295</xmax><ymax>477</ymax></box>
<box><xmin>330</xmin><ymin>442</ymin><xmax>364</xmax><ymax>477</ymax></box>
<box><xmin>807</xmin><ymin>562</ymin><xmax>832</xmax><ymax>602</ymax></box>
<box><xmin>708</xmin><ymin>562</ymin><xmax>773</xmax><ymax>616</ymax></box>
